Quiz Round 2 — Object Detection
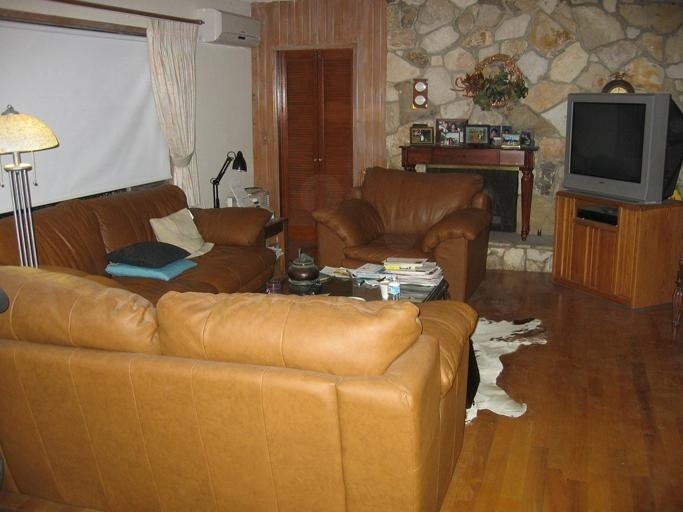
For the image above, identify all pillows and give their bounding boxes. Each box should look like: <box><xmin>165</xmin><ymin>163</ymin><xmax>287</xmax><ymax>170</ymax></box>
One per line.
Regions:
<box><xmin>104</xmin><ymin>207</ymin><xmax>214</xmax><ymax>283</ymax></box>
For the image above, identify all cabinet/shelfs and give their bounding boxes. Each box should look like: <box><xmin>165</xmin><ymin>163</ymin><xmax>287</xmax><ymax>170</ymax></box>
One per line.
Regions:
<box><xmin>550</xmin><ymin>190</ymin><xmax>682</xmax><ymax>310</ymax></box>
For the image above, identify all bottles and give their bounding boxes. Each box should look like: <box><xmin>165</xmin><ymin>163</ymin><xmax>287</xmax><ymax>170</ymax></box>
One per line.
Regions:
<box><xmin>386</xmin><ymin>273</ymin><xmax>401</xmax><ymax>302</ymax></box>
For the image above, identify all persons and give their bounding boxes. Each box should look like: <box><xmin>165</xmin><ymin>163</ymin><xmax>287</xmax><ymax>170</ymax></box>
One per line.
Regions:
<box><xmin>449</xmin><ymin>123</ymin><xmax>461</xmax><ymax>133</ymax></box>
<box><xmin>446</xmin><ymin>139</ymin><xmax>449</xmax><ymax>145</ymax></box>
<box><xmin>489</xmin><ymin>129</ymin><xmax>497</xmax><ymax>144</ymax></box>
<box><xmin>419</xmin><ymin>133</ymin><xmax>425</xmax><ymax>142</ymax></box>
<box><xmin>449</xmin><ymin>138</ymin><xmax>453</xmax><ymax>145</ymax></box>
<box><xmin>503</xmin><ymin>129</ymin><xmax>509</xmax><ymax>134</ymax></box>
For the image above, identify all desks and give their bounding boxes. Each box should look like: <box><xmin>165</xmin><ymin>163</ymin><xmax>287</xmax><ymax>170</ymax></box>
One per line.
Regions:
<box><xmin>398</xmin><ymin>145</ymin><xmax>539</xmax><ymax>242</ymax></box>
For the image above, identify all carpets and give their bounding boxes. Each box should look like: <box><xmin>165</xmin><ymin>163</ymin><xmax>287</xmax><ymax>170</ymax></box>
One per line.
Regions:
<box><xmin>463</xmin><ymin>316</ymin><xmax>547</xmax><ymax>426</ymax></box>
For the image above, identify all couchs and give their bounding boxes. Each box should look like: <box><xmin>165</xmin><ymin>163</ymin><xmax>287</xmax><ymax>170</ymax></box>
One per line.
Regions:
<box><xmin>0</xmin><ymin>179</ymin><xmax>479</xmax><ymax>511</ymax></box>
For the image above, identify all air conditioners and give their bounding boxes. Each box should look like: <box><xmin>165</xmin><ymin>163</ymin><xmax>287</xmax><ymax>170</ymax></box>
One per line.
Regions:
<box><xmin>198</xmin><ymin>8</ymin><xmax>261</xmax><ymax>49</ymax></box>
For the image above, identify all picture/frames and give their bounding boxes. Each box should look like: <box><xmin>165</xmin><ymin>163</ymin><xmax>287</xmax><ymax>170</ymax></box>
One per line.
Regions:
<box><xmin>409</xmin><ymin>118</ymin><xmax>534</xmax><ymax>146</ymax></box>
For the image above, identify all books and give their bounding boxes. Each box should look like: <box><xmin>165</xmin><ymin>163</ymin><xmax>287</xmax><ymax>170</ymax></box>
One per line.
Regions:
<box><xmin>350</xmin><ymin>256</ymin><xmax>443</xmax><ymax>287</ymax></box>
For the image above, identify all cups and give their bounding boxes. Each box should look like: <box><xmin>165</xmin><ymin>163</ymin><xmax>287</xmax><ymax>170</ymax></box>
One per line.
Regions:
<box><xmin>266</xmin><ymin>279</ymin><xmax>282</xmax><ymax>294</ymax></box>
<box><xmin>380</xmin><ymin>280</ymin><xmax>389</xmax><ymax>301</ymax></box>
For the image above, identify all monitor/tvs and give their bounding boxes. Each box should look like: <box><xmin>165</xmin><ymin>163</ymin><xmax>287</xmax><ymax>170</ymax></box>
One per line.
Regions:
<box><xmin>560</xmin><ymin>92</ymin><xmax>683</xmax><ymax>204</ymax></box>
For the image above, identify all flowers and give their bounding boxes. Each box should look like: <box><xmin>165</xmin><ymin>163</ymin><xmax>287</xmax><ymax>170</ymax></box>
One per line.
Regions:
<box><xmin>462</xmin><ymin>60</ymin><xmax>528</xmax><ymax>113</ymax></box>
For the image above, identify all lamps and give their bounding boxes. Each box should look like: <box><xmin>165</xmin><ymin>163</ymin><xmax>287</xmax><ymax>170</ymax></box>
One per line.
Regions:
<box><xmin>208</xmin><ymin>150</ymin><xmax>247</xmax><ymax>208</ymax></box>
<box><xmin>0</xmin><ymin>105</ymin><xmax>58</xmax><ymax>268</ymax></box>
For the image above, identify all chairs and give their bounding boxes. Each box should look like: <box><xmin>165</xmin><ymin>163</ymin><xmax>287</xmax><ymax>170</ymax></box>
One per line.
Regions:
<box><xmin>309</xmin><ymin>166</ymin><xmax>492</xmax><ymax>303</ymax></box>
